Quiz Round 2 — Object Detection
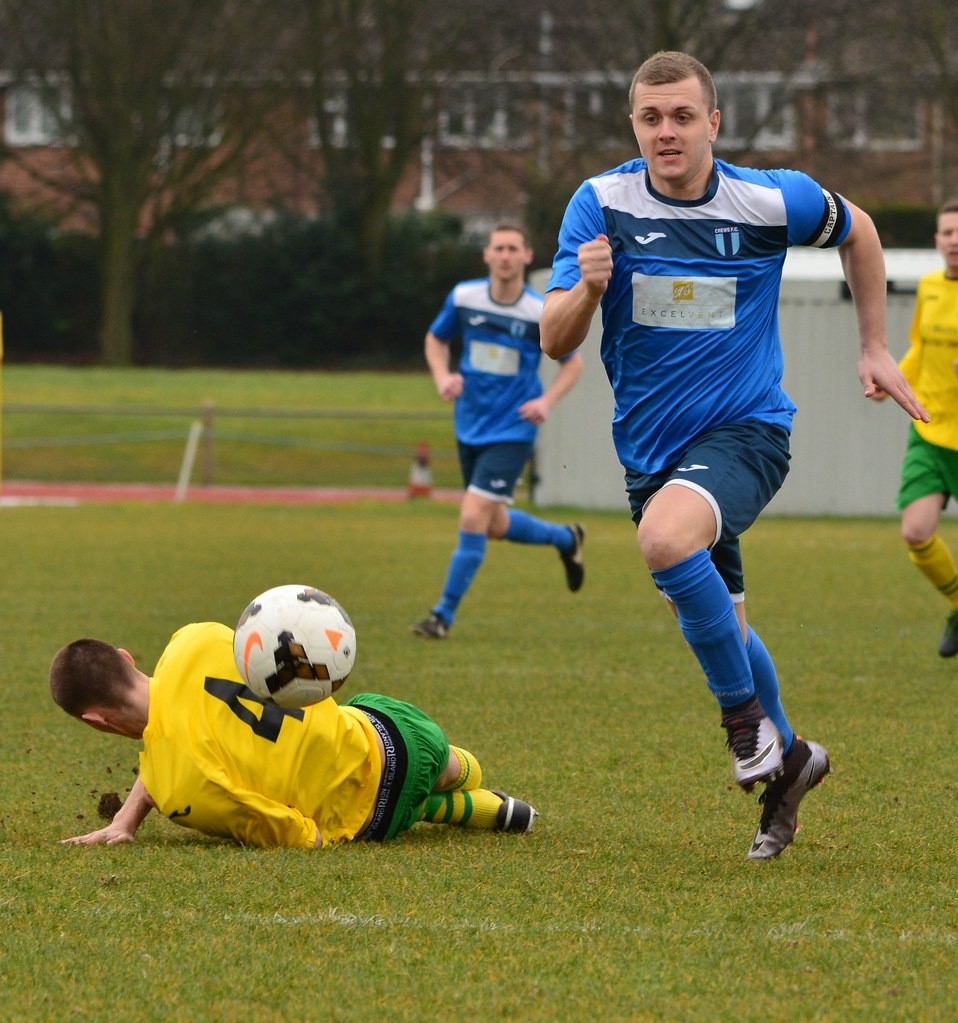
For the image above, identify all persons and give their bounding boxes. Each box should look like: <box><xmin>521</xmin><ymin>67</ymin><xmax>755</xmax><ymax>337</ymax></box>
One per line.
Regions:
<box><xmin>422</xmin><ymin>217</ymin><xmax>586</xmax><ymax>644</ymax></box>
<box><xmin>529</xmin><ymin>49</ymin><xmax>930</xmax><ymax>859</ymax></box>
<box><xmin>47</xmin><ymin>621</ymin><xmax>539</xmax><ymax>853</ymax></box>
<box><xmin>871</xmin><ymin>199</ymin><xmax>958</xmax><ymax>657</ymax></box>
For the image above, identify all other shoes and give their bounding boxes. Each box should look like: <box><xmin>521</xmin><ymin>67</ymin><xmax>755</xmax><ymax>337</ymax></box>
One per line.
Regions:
<box><xmin>938</xmin><ymin>612</ymin><xmax>958</xmax><ymax>656</ymax></box>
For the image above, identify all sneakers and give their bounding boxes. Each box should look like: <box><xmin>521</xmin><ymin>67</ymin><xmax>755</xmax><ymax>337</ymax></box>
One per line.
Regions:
<box><xmin>492</xmin><ymin>789</ymin><xmax>540</xmax><ymax>835</ymax></box>
<box><xmin>722</xmin><ymin>709</ymin><xmax>784</xmax><ymax>793</ymax></box>
<box><xmin>412</xmin><ymin>616</ymin><xmax>446</xmax><ymax>640</ymax></box>
<box><xmin>559</xmin><ymin>524</ymin><xmax>586</xmax><ymax>591</ymax></box>
<box><xmin>747</xmin><ymin>736</ymin><xmax>834</xmax><ymax>861</ymax></box>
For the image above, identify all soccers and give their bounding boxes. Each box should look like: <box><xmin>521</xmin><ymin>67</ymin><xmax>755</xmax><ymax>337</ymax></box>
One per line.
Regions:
<box><xmin>232</xmin><ymin>584</ymin><xmax>358</xmax><ymax>709</ymax></box>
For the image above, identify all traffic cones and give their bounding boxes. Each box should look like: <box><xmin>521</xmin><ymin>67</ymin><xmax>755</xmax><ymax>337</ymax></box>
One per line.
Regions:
<box><xmin>404</xmin><ymin>439</ymin><xmax>433</xmax><ymax>504</ymax></box>
